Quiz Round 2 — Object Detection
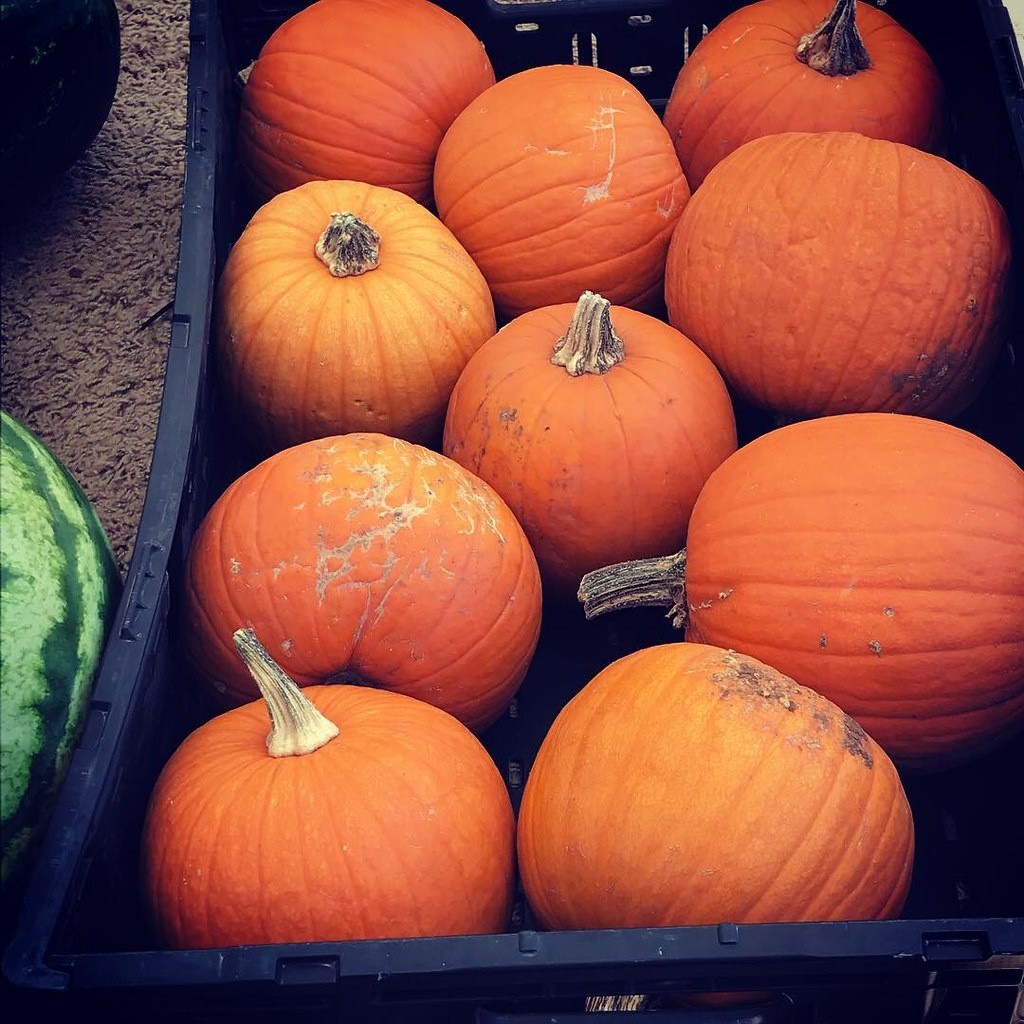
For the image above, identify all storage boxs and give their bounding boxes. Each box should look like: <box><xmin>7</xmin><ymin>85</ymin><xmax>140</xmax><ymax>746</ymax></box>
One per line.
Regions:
<box><xmin>2</xmin><ymin>0</ymin><xmax>1024</xmax><ymax>1023</ymax></box>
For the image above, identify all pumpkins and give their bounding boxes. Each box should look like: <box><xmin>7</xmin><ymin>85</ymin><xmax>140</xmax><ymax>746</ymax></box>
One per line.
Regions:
<box><xmin>148</xmin><ymin>0</ymin><xmax>1024</xmax><ymax>1003</ymax></box>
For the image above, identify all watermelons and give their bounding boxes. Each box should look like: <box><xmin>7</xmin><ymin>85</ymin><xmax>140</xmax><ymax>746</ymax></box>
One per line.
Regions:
<box><xmin>0</xmin><ymin>405</ymin><xmax>125</xmax><ymax>895</ymax></box>
